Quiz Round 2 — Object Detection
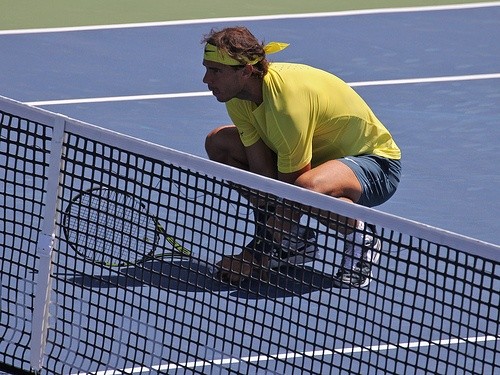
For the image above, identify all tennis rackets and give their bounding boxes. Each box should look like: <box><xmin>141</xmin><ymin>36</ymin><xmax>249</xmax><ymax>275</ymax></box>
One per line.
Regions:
<box><xmin>63</xmin><ymin>188</ymin><xmax>250</xmax><ymax>288</ymax></box>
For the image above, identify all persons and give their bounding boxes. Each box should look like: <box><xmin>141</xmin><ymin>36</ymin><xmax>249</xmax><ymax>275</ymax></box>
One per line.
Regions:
<box><xmin>200</xmin><ymin>25</ymin><xmax>402</xmax><ymax>289</ymax></box>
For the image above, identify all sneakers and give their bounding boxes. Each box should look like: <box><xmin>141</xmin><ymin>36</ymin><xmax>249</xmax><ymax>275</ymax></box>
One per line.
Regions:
<box><xmin>262</xmin><ymin>228</ymin><xmax>320</xmax><ymax>266</ymax></box>
<box><xmin>334</xmin><ymin>219</ymin><xmax>380</xmax><ymax>290</ymax></box>
